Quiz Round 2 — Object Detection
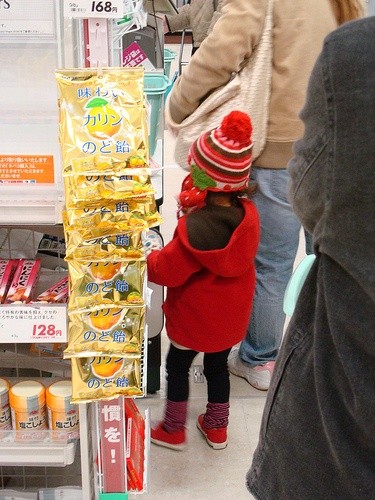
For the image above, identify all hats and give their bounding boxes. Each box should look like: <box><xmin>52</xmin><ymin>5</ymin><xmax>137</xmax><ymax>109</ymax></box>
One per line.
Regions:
<box><xmin>176</xmin><ymin>109</ymin><xmax>253</xmax><ymax>218</ymax></box>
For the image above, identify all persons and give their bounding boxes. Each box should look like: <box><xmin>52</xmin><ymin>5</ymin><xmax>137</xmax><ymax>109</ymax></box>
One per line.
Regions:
<box><xmin>245</xmin><ymin>14</ymin><xmax>375</xmax><ymax>500</ymax></box>
<box><xmin>146</xmin><ymin>111</ymin><xmax>260</xmax><ymax>453</ymax></box>
<box><xmin>164</xmin><ymin>0</ymin><xmax>365</xmax><ymax>390</ymax></box>
<box><xmin>163</xmin><ymin>0</ymin><xmax>223</xmax><ymax>56</ymax></box>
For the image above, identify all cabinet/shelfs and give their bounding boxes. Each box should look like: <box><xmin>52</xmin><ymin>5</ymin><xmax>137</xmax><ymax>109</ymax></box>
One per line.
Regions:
<box><xmin>0</xmin><ymin>0</ymin><xmax>96</xmax><ymax>500</ymax></box>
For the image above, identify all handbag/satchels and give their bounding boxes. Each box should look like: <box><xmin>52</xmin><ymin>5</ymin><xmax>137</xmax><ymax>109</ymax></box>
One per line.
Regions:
<box><xmin>174</xmin><ymin>0</ymin><xmax>273</xmax><ymax>172</ymax></box>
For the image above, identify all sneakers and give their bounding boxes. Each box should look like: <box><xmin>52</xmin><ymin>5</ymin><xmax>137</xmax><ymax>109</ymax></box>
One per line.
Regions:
<box><xmin>150</xmin><ymin>420</ymin><xmax>185</xmax><ymax>450</ymax></box>
<box><xmin>227</xmin><ymin>345</ymin><xmax>276</xmax><ymax>390</ymax></box>
<box><xmin>197</xmin><ymin>414</ymin><xmax>228</xmax><ymax>450</ymax></box>
